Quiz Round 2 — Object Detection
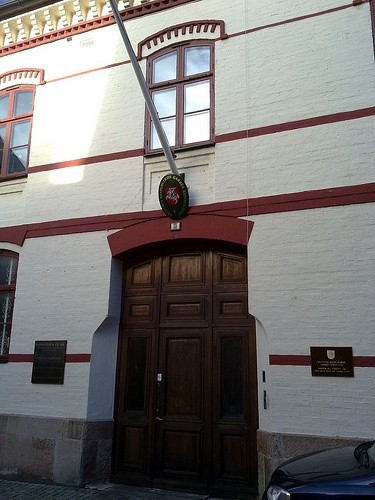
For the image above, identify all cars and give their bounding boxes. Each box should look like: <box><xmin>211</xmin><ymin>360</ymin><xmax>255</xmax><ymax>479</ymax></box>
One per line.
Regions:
<box><xmin>260</xmin><ymin>437</ymin><xmax>375</xmax><ymax>500</ymax></box>
